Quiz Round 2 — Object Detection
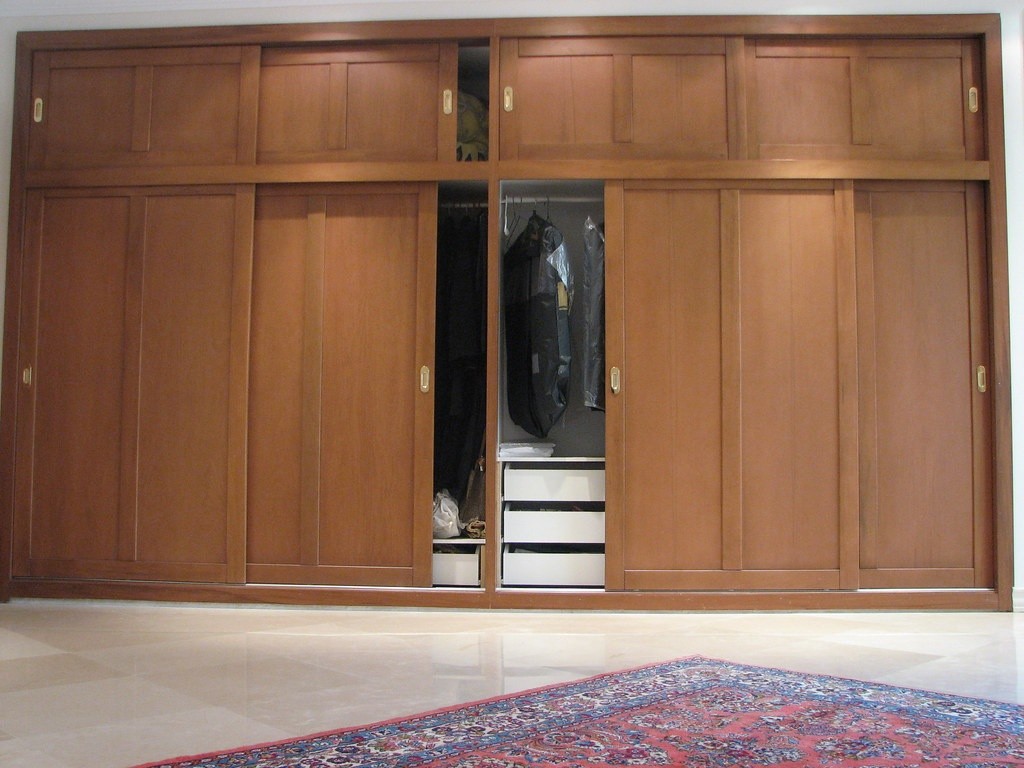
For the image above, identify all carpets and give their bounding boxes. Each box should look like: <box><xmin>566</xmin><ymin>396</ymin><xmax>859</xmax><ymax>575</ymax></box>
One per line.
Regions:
<box><xmin>125</xmin><ymin>656</ymin><xmax>1024</xmax><ymax>768</ymax></box>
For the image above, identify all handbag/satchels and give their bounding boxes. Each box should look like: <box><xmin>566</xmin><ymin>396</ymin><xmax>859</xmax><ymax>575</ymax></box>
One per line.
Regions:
<box><xmin>432</xmin><ymin>490</ymin><xmax>468</xmax><ymax>538</ymax></box>
<box><xmin>460</xmin><ymin>429</ymin><xmax>487</xmax><ymax>539</ymax></box>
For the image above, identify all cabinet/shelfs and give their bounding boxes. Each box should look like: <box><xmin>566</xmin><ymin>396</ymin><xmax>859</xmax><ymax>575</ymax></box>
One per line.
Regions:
<box><xmin>0</xmin><ymin>15</ymin><xmax>1014</xmax><ymax>612</ymax></box>
